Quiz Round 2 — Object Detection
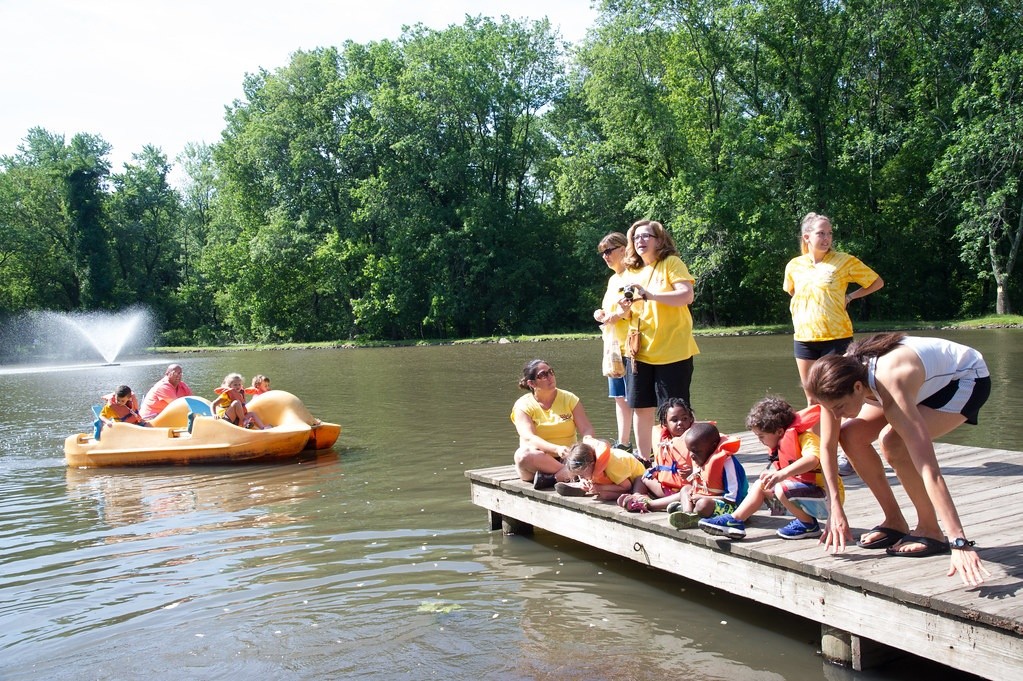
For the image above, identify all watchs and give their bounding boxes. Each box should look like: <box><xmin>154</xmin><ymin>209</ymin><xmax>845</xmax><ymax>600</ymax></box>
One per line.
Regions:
<box><xmin>949</xmin><ymin>538</ymin><xmax>976</xmax><ymax>550</ymax></box>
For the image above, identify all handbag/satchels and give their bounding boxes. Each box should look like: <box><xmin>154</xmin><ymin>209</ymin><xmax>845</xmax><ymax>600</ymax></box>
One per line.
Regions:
<box><xmin>629</xmin><ymin>333</ymin><xmax>640</xmax><ymax>374</ymax></box>
<box><xmin>599</xmin><ymin>321</ymin><xmax>626</xmax><ymax>378</ymax></box>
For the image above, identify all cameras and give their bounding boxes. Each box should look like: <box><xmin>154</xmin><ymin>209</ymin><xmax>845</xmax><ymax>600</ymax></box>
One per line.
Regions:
<box><xmin>618</xmin><ymin>285</ymin><xmax>635</xmax><ymax>299</ymax></box>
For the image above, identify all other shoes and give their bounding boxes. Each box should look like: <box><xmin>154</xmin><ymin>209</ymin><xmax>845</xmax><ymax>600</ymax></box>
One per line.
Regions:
<box><xmin>534</xmin><ymin>471</ymin><xmax>554</xmax><ymax>490</ymax></box>
<box><xmin>554</xmin><ymin>481</ymin><xmax>586</xmax><ymax>497</ymax></box>
<box><xmin>615</xmin><ymin>493</ymin><xmax>649</xmax><ymax>514</ymax></box>
<box><xmin>667</xmin><ymin>504</ymin><xmax>681</xmax><ymax>514</ymax></box>
<box><xmin>670</xmin><ymin>511</ymin><xmax>703</xmax><ymax>530</ymax></box>
<box><xmin>247</xmin><ymin>417</ymin><xmax>255</xmax><ymax>429</ymax></box>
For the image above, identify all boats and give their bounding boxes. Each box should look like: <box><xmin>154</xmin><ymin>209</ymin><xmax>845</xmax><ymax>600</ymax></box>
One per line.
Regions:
<box><xmin>64</xmin><ymin>390</ymin><xmax>342</xmax><ymax>469</ymax></box>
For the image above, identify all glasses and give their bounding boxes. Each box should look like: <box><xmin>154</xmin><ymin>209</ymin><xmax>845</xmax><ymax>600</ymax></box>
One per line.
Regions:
<box><xmin>533</xmin><ymin>368</ymin><xmax>555</xmax><ymax>382</ymax></box>
<box><xmin>631</xmin><ymin>233</ymin><xmax>659</xmax><ymax>242</ymax></box>
<box><xmin>600</xmin><ymin>245</ymin><xmax>622</xmax><ymax>258</ymax></box>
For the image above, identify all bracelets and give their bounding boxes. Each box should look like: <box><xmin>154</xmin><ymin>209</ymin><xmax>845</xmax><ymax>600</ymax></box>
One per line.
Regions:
<box><xmin>844</xmin><ymin>294</ymin><xmax>852</xmax><ymax>303</ymax></box>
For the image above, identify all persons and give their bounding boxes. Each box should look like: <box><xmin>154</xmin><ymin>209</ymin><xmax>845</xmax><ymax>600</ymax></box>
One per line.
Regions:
<box><xmin>211</xmin><ymin>372</ymin><xmax>273</xmax><ymax>431</ymax></box>
<box><xmin>615</xmin><ymin>219</ymin><xmax>700</xmax><ymax>469</ymax></box>
<box><xmin>805</xmin><ymin>331</ymin><xmax>992</xmax><ymax>589</ymax></box>
<box><xmin>245</xmin><ymin>375</ymin><xmax>272</xmax><ymax>393</ymax></box>
<box><xmin>138</xmin><ymin>364</ymin><xmax>192</xmax><ymax>422</ymax></box>
<box><xmin>782</xmin><ymin>211</ymin><xmax>883</xmax><ymax>476</ymax></box>
<box><xmin>99</xmin><ymin>385</ymin><xmax>153</xmax><ymax>428</ymax></box>
<box><xmin>697</xmin><ymin>398</ymin><xmax>846</xmax><ymax>541</ymax></box>
<box><xmin>593</xmin><ymin>232</ymin><xmax>655</xmax><ymax>462</ymax></box>
<box><xmin>510</xmin><ymin>359</ymin><xmax>752</xmax><ymax>529</ymax></box>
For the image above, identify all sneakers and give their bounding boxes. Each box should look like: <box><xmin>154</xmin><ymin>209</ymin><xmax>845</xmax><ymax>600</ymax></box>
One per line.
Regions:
<box><xmin>613</xmin><ymin>440</ymin><xmax>632</xmax><ymax>453</ymax></box>
<box><xmin>698</xmin><ymin>513</ymin><xmax>746</xmax><ymax>540</ymax></box>
<box><xmin>835</xmin><ymin>453</ymin><xmax>857</xmax><ymax>476</ymax></box>
<box><xmin>634</xmin><ymin>447</ymin><xmax>654</xmax><ymax>468</ymax></box>
<box><xmin>778</xmin><ymin>517</ymin><xmax>821</xmax><ymax>539</ymax></box>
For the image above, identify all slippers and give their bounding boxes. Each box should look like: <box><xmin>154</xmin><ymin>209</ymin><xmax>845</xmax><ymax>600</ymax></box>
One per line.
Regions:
<box><xmin>887</xmin><ymin>532</ymin><xmax>950</xmax><ymax>556</ymax></box>
<box><xmin>856</xmin><ymin>522</ymin><xmax>906</xmax><ymax>548</ymax></box>
